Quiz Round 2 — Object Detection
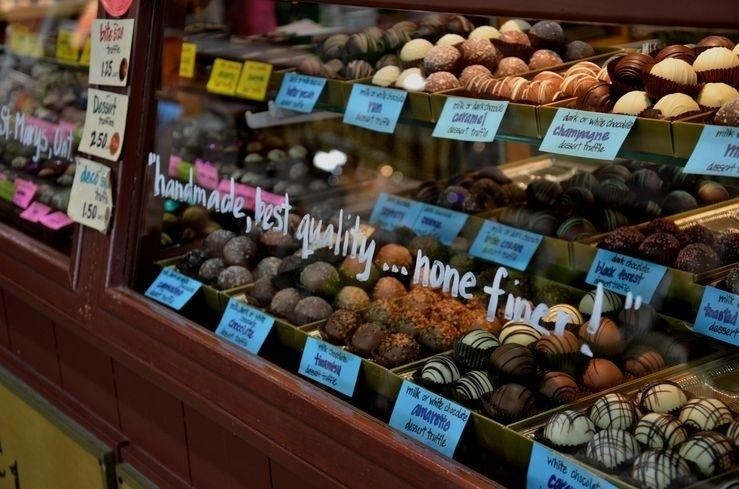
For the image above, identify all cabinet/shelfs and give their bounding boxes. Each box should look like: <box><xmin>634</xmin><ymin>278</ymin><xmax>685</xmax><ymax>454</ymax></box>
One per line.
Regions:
<box><xmin>1</xmin><ymin>1</ymin><xmax>738</xmax><ymax>487</ymax></box>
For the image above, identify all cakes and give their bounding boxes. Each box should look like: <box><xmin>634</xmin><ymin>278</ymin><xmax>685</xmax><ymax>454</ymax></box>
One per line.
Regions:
<box><xmin>597</xmin><ymin>217</ymin><xmax>738</xmax><ymax>292</ymax></box>
<box><xmin>2</xmin><ymin>53</ymin><xmax>89</xmax><ymax>144</ymax></box>
<box><xmin>411</xmin><ymin>165</ymin><xmax>528</xmax><ymax>215</ymax></box>
<box><xmin>497</xmin><ymin>159</ymin><xmax>738</xmax><ymax>241</ymax></box>
<box><xmin>2</xmin><ymin>134</ymin><xmax>76</xmax><ymax>210</ymax></box>
<box><xmin>295</xmin><ymin>13</ymin><xmax>475</xmax><ymax>80</ymax></box>
<box><xmin>535</xmin><ymin>379</ymin><xmax>739</xmax><ymax>489</ymax></box>
<box><xmin>464</xmin><ymin>60</ymin><xmax>611</xmax><ymax>105</ymax></box>
<box><xmin>412</xmin><ymin>288</ymin><xmax>663</xmax><ymax>426</ymax></box>
<box><xmin>171</xmin><ymin>121</ymin><xmax>360</xmax><ymax>197</ymax></box>
<box><xmin>185</xmin><ymin>17</ymin><xmax>344</xmax><ymax>71</ymax></box>
<box><xmin>566</xmin><ymin>36</ymin><xmax>738</xmax><ymax>126</ymax></box>
<box><xmin>369</xmin><ymin>18</ymin><xmax>596</xmax><ymax>94</ymax></box>
<box><xmin>246</xmin><ymin>226</ymin><xmax>480</xmax><ymax>326</ymax></box>
<box><xmin>175</xmin><ymin>200</ymin><xmax>361</xmax><ymax>291</ymax></box>
<box><xmin>317</xmin><ymin>268</ymin><xmax>571</xmax><ymax>368</ymax></box>
